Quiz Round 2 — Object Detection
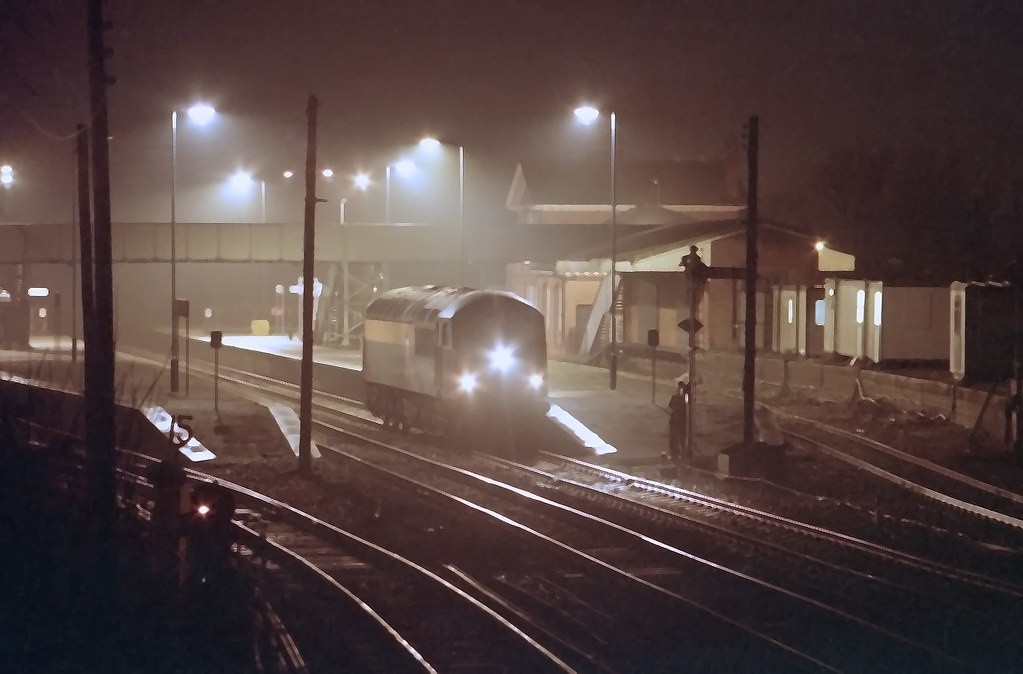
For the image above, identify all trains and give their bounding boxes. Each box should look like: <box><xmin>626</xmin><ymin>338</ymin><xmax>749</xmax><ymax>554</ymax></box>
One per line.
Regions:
<box><xmin>356</xmin><ymin>284</ymin><xmax>551</xmax><ymax>448</ymax></box>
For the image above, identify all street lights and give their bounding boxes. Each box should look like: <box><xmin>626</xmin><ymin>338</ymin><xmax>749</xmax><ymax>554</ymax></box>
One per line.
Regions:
<box><xmin>573</xmin><ymin>106</ymin><xmax>621</xmax><ymax>389</ymax></box>
<box><xmin>420</xmin><ymin>137</ymin><xmax>466</xmax><ymax>281</ymax></box>
<box><xmin>262</xmin><ymin>168</ymin><xmax>293</xmax><ymax>223</ymax></box>
<box><xmin>169</xmin><ymin>102</ymin><xmax>215</xmax><ymax>391</ymax></box>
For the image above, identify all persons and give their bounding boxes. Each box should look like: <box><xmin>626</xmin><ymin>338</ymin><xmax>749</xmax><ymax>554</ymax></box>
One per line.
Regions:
<box><xmin>667</xmin><ymin>381</ymin><xmax>686</xmax><ymax>460</ymax></box>
